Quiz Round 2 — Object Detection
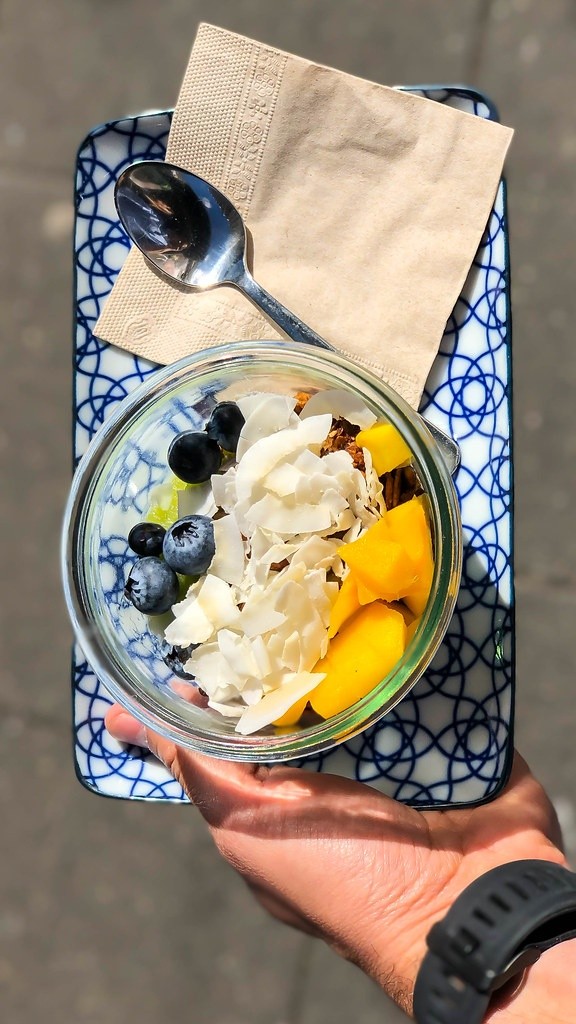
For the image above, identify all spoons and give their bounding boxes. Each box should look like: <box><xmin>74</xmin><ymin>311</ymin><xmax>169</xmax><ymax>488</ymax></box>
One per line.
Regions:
<box><xmin>115</xmin><ymin>161</ymin><xmax>462</xmax><ymax>475</ymax></box>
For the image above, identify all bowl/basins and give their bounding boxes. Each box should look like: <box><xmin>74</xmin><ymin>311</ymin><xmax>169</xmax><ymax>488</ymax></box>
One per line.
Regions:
<box><xmin>63</xmin><ymin>338</ymin><xmax>459</xmax><ymax>762</ymax></box>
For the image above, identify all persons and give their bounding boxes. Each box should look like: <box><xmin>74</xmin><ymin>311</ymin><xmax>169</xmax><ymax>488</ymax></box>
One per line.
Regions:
<box><xmin>104</xmin><ymin>681</ymin><xmax>576</xmax><ymax>1024</ymax></box>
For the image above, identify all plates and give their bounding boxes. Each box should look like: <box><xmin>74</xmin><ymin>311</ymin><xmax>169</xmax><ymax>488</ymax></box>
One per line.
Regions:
<box><xmin>71</xmin><ymin>86</ymin><xmax>517</xmax><ymax>809</ymax></box>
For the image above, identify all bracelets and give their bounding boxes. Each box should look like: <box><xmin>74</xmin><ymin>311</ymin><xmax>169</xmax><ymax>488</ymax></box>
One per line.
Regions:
<box><xmin>411</xmin><ymin>859</ymin><xmax>576</xmax><ymax>1024</ymax></box>
